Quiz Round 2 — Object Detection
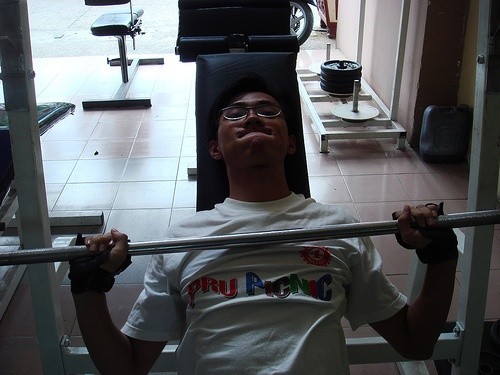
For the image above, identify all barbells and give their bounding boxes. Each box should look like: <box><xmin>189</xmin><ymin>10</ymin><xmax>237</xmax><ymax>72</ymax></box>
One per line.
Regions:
<box><xmin>0</xmin><ymin>208</ymin><xmax>500</xmax><ymax>267</ymax></box>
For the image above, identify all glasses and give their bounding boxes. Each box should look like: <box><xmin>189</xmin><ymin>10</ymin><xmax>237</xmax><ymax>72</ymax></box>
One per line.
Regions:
<box><xmin>216</xmin><ymin>101</ymin><xmax>291</xmax><ymax>144</ymax></box>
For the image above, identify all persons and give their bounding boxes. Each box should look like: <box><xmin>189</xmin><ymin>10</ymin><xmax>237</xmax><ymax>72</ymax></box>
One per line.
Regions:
<box><xmin>70</xmin><ymin>76</ymin><xmax>459</xmax><ymax>375</ymax></box>
